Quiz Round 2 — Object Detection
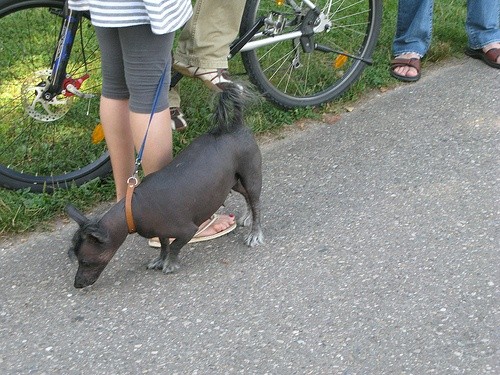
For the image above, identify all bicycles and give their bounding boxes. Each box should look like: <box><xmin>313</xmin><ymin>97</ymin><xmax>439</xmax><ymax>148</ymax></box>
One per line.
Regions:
<box><xmin>0</xmin><ymin>0</ymin><xmax>383</xmax><ymax>195</ymax></box>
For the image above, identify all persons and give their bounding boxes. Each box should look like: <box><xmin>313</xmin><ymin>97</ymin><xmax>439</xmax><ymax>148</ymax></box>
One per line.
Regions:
<box><xmin>67</xmin><ymin>0</ymin><xmax>237</xmax><ymax>248</ymax></box>
<box><xmin>389</xmin><ymin>0</ymin><xmax>500</xmax><ymax>82</ymax></box>
<box><xmin>168</xmin><ymin>0</ymin><xmax>247</xmax><ymax>131</ymax></box>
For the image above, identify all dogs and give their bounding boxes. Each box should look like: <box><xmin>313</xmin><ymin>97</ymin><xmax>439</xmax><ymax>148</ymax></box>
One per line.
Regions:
<box><xmin>68</xmin><ymin>78</ymin><xmax>268</xmax><ymax>289</ymax></box>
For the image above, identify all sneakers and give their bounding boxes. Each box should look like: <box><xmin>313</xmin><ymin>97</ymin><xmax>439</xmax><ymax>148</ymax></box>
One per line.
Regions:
<box><xmin>169</xmin><ymin>108</ymin><xmax>188</xmax><ymax>130</ymax></box>
<box><xmin>173</xmin><ymin>57</ymin><xmax>243</xmax><ymax>94</ymax></box>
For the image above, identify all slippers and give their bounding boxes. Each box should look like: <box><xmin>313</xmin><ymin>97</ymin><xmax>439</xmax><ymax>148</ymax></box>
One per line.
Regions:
<box><xmin>148</xmin><ymin>213</ymin><xmax>237</xmax><ymax>247</ymax></box>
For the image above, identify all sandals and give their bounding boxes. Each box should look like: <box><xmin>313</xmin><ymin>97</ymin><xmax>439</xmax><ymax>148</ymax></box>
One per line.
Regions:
<box><xmin>389</xmin><ymin>53</ymin><xmax>421</xmax><ymax>80</ymax></box>
<box><xmin>465</xmin><ymin>44</ymin><xmax>500</xmax><ymax>69</ymax></box>
